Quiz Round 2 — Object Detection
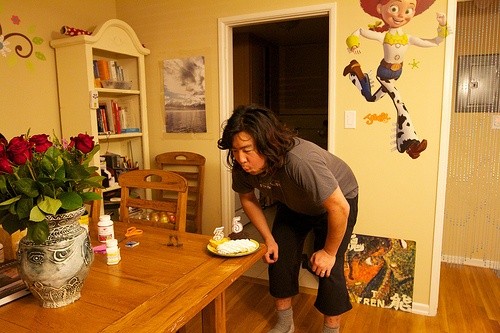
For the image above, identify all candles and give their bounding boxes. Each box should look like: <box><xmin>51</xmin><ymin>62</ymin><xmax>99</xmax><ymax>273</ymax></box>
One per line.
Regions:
<box><xmin>232</xmin><ymin>216</ymin><xmax>243</xmax><ymax>234</ymax></box>
<box><xmin>213</xmin><ymin>225</ymin><xmax>224</xmax><ymax>241</ymax></box>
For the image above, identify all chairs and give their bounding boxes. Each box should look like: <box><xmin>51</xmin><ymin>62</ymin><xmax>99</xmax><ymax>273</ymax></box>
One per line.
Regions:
<box><xmin>155</xmin><ymin>153</ymin><xmax>205</xmax><ymax>234</ymax></box>
<box><xmin>119</xmin><ymin>170</ymin><xmax>190</xmax><ymax>333</ymax></box>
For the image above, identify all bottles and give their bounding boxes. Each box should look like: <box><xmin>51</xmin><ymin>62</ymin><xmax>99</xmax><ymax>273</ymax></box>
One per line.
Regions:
<box><xmin>106</xmin><ymin>239</ymin><xmax>122</xmax><ymax>265</ymax></box>
<box><xmin>97</xmin><ymin>214</ymin><xmax>114</xmax><ymax>243</ymax></box>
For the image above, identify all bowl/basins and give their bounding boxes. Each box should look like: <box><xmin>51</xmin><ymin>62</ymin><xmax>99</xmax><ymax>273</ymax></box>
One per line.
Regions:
<box><xmin>102</xmin><ymin>80</ymin><xmax>132</xmax><ymax>89</ymax></box>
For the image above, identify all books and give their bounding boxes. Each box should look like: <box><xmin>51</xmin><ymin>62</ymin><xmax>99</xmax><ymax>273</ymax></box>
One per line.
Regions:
<box><xmin>0</xmin><ymin>259</ymin><xmax>32</xmax><ymax>306</ymax></box>
<box><xmin>101</xmin><ymin>153</ymin><xmax>125</xmax><ymax>170</ymax></box>
<box><xmin>97</xmin><ymin>100</ymin><xmax>141</xmax><ymax>134</ymax></box>
<box><xmin>93</xmin><ymin>59</ymin><xmax>132</xmax><ymax>88</ymax></box>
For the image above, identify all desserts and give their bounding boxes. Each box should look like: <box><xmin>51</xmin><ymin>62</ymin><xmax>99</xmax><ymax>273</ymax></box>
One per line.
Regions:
<box><xmin>209</xmin><ymin>230</ymin><xmax>256</xmax><ymax>254</ymax></box>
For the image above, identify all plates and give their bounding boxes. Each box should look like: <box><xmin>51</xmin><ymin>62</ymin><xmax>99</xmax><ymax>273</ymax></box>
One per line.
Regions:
<box><xmin>207</xmin><ymin>239</ymin><xmax>260</xmax><ymax>257</ymax></box>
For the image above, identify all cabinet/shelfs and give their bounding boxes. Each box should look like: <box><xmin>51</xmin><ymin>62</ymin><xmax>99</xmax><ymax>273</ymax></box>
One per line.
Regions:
<box><xmin>233</xmin><ymin>33</ymin><xmax>279</xmax><ymax>114</ymax></box>
<box><xmin>49</xmin><ymin>18</ymin><xmax>152</xmax><ymax>221</ymax></box>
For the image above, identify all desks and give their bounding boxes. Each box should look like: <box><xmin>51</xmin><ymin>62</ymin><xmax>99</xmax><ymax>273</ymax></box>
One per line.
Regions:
<box><xmin>0</xmin><ymin>219</ymin><xmax>267</xmax><ymax>332</ymax></box>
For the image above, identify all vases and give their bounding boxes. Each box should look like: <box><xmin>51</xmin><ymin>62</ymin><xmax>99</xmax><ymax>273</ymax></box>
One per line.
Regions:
<box><xmin>15</xmin><ymin>206</ymin><xmax>95</xmax><ymax>308</ymax></box>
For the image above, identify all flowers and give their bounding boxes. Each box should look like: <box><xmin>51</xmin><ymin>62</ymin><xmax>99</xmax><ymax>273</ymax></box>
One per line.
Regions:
<box><xmin>0</xmin><ymin>128</ymin><xmax>107</xmax><ymax>245</ymax></box>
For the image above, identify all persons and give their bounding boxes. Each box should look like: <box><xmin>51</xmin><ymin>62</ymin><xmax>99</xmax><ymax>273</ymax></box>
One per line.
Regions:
<box><xmin>217</xmin><ymin>102</ymin><xmax>360</xmax><ymax>333</ymax></box>
